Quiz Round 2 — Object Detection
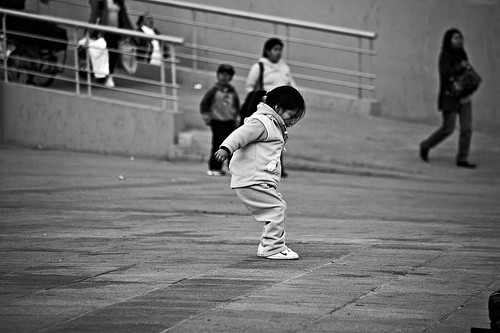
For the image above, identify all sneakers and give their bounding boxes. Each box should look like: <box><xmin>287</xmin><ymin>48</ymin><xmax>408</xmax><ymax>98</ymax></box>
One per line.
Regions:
<box><xmin>257</xmin><ymin>244</ymin><xmax>266</xmax><ymax>257</ymax></box>
<box><xmin>267</xmin><ymin>248</ymin><xmax>298</xmax><ymax>260</ymax></box>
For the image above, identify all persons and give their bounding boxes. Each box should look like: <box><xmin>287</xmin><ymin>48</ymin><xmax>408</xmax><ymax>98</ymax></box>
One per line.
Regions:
<box><xmin>247</xmin><ymin>38</ymin><xmax>299</xmax><ymax>177</ymax></box>
<box><xmin>200</xmin><ymin>63</ymin><xmax>240</xmax><ymax>175</ymax></box>
<box><xmin>215</xmin><ymin>85</ymin><xmax>306</xmax><ymax>259</ymax></box>
<box><xmin>131</xmin><ymin>12</ymin><xmax>170</xmax><ymax>66</ymax></box>
<box><xmin>419</xmin><ymin>28</ymin><xmax>481</xmax><ymax>168</ymax></box>
<box><xmin>87</xmin><ymin>0</ymin><xmax>134</xmax><ymax>87</ymax></box>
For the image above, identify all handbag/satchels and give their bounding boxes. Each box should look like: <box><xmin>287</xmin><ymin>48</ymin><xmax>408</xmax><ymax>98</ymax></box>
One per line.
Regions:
<box><xmin>76</xmin><ymin>27</ymin><xmax>110</xmax><ymax>80</ymax></box>
<box><xmin>118</xmin><ymin>33</ymin><xmax>153</xmax><ymax>74</ymax></box>
<box><xmin>241</xmin><ymin>62</ymin><xmax>266</xmax><ymax>116</ymax></box>
<box><xmin>448</xmin><ymin>60</ymin><xmax>481</xmax><ymax>97</ymax></box>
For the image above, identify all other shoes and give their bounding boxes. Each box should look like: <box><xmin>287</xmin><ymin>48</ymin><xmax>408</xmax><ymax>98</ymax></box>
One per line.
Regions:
<box><xmin>419</xmin><ymin>143</ymin><xmax>428</xmax><ymax>161</ymax></box>
<box><xmin>458</xmin><ymin>160</ymin><xmax>475</xmax><ymax>167</ymax></box>
<box><xmin>208</xmin><ymin>169</ymin><xmax>224</xmax><ymax>176</ymax></box>
